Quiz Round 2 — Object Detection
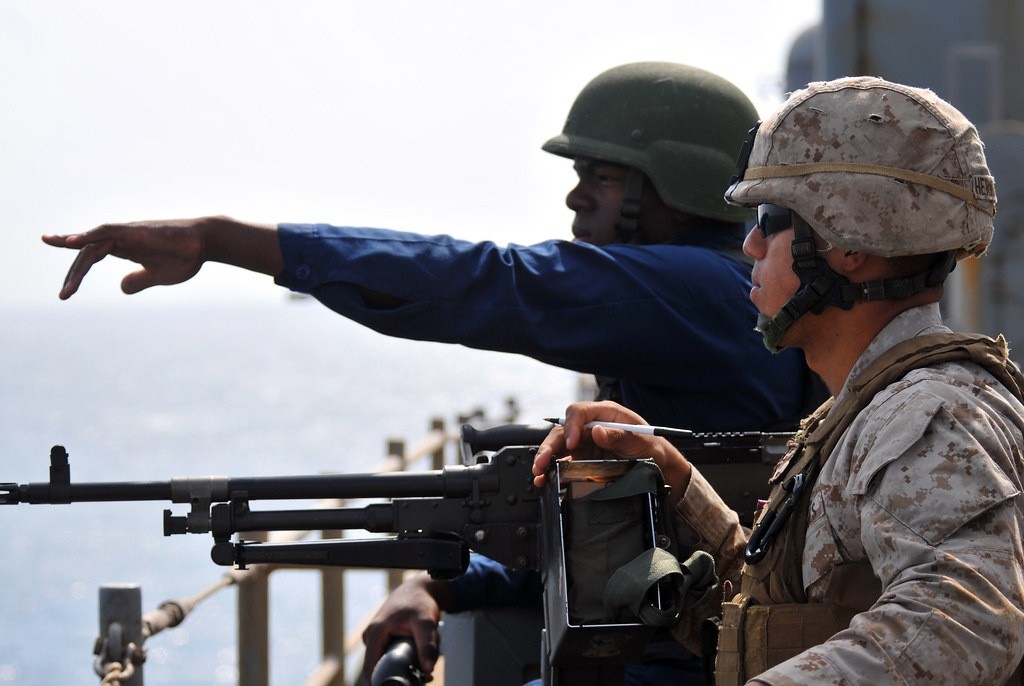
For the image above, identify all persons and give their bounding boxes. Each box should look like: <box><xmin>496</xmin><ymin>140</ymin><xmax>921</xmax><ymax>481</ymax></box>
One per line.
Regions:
<box><xmin>43</xmin><ymin>61</ymin><xmax>1024</xmax><ymax>686</ymax></box>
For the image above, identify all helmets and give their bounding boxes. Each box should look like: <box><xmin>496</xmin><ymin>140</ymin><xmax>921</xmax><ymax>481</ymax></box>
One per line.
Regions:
<box><xmin>724</xmin><ymin>77</ymin><xmax>996</xmax><ymax>262</ymax></box>
<box><xmin>542</xmin><ymin>62</ymin><xmax>761</xmax><ymax>221</ymax></box>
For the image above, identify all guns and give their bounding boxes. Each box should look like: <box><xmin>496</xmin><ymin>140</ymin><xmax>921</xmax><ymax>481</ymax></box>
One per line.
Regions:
<box><xmin>0</xmin><ymin>424</ymin><xmax>803</xmax><ymax>684</ymax></box>
